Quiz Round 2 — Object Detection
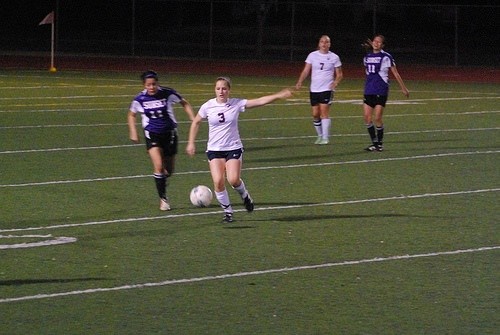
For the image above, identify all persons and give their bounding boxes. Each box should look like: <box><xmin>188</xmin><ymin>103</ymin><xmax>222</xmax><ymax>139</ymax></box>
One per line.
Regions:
<box><xmin>127</xmin><ymin>71</ymin><xmax>195</xmax><ymax>211</ymax></box>
<box><xmin>363</xmin><ymin>36</ymin><xmax>410</xmax><ymax>152</ymax></box>
<box><xmin>295</xmin><ymin>35</ymin><xmax>342</xmax><ymax>145</ymax></box>
<box><xmin>186</xmin><ymin>76</ymin><xmax>294</xmax><ymax>223</ymax></box>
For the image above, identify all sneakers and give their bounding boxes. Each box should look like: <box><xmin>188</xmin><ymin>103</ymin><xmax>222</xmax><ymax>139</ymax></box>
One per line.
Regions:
<box><xmin>160</xmin><ymin>198</ymin><xmax>171</xmax><ymax>211</ymax></box>
<box><xmin>242</xmin><ymin>194</ymin><xmax>254</xmax><ymax>212</ymax></box>
<box><xmin>221</xmin><ymin>212</ymin><xmax>233</xmax><ymax>224</ymax></box>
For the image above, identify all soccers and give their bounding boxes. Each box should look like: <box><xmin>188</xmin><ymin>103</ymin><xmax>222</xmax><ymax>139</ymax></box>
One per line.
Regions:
<box><xmin>189</xmin><ymin>185</ymin><xmax>213</xmax><ymax>208</ymax></box>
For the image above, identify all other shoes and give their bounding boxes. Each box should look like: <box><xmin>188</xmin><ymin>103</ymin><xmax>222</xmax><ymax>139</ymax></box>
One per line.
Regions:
<box><xmin>367</xmin><ymin>146</ymin><xmax>377</xmax><ymax>151</ymax></box>
<box><xmin>378</xmin><ymin>145</ymin><xmax>384</xmax><ymax>152</ymax></box>
<box><xmin>314</xmin><ymin>137</ymin><xmax>329</xmax><ymax>145</ymax></box>
